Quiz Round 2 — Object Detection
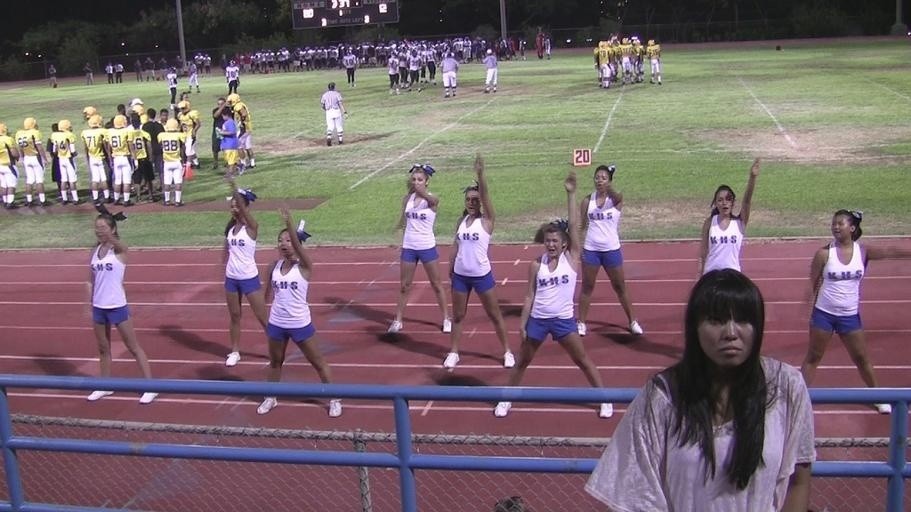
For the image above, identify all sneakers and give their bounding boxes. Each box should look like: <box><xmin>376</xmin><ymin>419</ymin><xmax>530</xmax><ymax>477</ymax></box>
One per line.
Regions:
<box><xmin>382</xmin><ymin>314</ymin><xmax>645</xmax><ymax>372</ymax></box>
<box><xmin>326</xmin><ymin>397</ymin><xmax>344</xmax><ymax>420</ymax></box>
<box><xmin>597</xmin><ymin>402</ymin><xmax>615</xmax><ymax>420</ymax></box>
<box><xmin>493</xmin><ymin>400</ymin><xmax>514</xmax><ymax>420</ymax></box>
<box><xmin>84</xmin><ymin>388</ymin><xmax>114</xmax><ymax>403</ymax></box>
<box><xmin>0</xmin><ymin>163</ymin><xmax>257</xmax><ymax>210</ymax></box>
<box><xmin>597</xmin><ymin>75</ymin><xmax>664</xmax><ymax>89</ymax></box>
<box><xmin>138</xmin><ymin>392</ymin><xmax>159</xmax><ymax>406</ymax></box>
<box><xmin>326</xmin><ymin>138</ymin><xmax>332</xmax><ymax>146</ymax></box>
<box><xmin>254</xmin><ymin>395</ymin><xmax>280</xmax><ymax>417</ymax></box>
<box><xmin>387</xmin><ymin>79</ymin><xmax>499</xmax><ymax>100</ymax></box>
<box><xmin>870</xmin><ymin>397</ymin><xmax>897</xmax><ymax>416</ymax></box>
<box><xmin>223</xmin><ymin>351</ymin><xmax>244</xmax><ymax>367</ymax></box>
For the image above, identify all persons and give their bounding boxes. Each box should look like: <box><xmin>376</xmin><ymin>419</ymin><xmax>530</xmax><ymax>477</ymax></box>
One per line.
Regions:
<box><xmin>187</xmin><ymin>59</ymin><xmax>201</xmax><ymax>94</ymax></box>
<box><xmin>584</xmin><ymin>269</ymin><xmax>818</xmax><ymax>512</ymax></box>
<box><xmin>114</xmin><ymin>60</ymin><xmax>124</xmax><ymax>84</ymax></box>
<box><xmin>443</xmin><ymin>151</ymin><xmax>516</xmax><ymax>371</ymax></box>
<box><xmin>494</xmin><ymin>169</ymin><xmax>614</xmax><ymax>418</ymax></box>
<box><xmin>167</xmin><ymin>66</ymin><xmax>179</xmax><ymax>110</ymax></box>
<box><xmin>48</xmin><ymin>63</ymin><xmax>58</xmax><ymax>88</ymax></box>
<box><xmin>801</xmin><ymin>209</ymin><xmax>911</xmax><ymax>413</ymax></box>
<box><xmin>321</xmin><ymin>82</ymin><xmax>349</xmax><ymax>147</ymax></box>
<box><xmin>86</xmin><ymin>204</ymin><xmax>160</xmax><ymax>403</ymax></box>
<box><xmin>693</xmin><ymin>156</ymin><xmax>760</xmax><ymax>276</ymax></box>
<box><xmin>593</xmin><ymin>33</ymin><xmax>662</xmax><ymax>89</ymax></box>
<box><xmin>257</xmin><ymin>202</ymin><xmax>344</xmax><ymax>417</ymax></box>
<box><xmin>83</xmin><ymin>61</ymin><xmax>95</xmax><ymax>85</ymax></box>
<box><xmin>1</xmin><ymin>91</ymin><xmax>256</xmax><ymax>207</ymax></box>
<box><xmin>386</xmin><ymin>164</ymin><xmax>452</xmax><ymax>334</ymax></box>
<box><xmin>135</xmin><ymin>52</ymin><xmax>212</xmax><ymax>82</ymax></box>
<box><xmin>575</xmin><ymin>166</ymin><xmax>645</xmax><ymax>337</ymax></box>
<box><xmin>105</xmin><ymin>61</ymin><xmax>114</xmax><ymax>84</ymax></box>
<box><xmin>223</xmin><ymin>171</ymin><xmax>288</xmax><ymax>367</ymax></box>
<box><xmin>544</xmin><ymin>35</ymin><xmax>553</xmax><ymax>60</ymax></box>
<box><xmin>536</xmin><ymin>27</ymin><xmax>545</xmax><ymax>60</ymax></box>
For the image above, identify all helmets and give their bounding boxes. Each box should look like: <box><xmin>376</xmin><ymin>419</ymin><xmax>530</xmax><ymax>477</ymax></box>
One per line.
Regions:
<box><xmin>163</xmin><ymin>118</ymin><xmax>181</xmax><ymax>132</ymax></box>
<box><xmin>87</xmin><ymin>114</ymin><xmax>105</xmax><ymax>128</ymax></box>
<box><xmin>110</xmin><ymin>98</ymin><xmax>144</xmax><ymax>128</ymax></box>
<box><xmin>56</xmin><ymin>119</ymin><xmax>74</xmax><ymax>131</ymax></box>
<box><xmin>0</xmin><ymin>123</ymin><xmax>11</xmax><ymax>136</ymax></box>
<box><xmin>83</xmin><ymin>106</ymin><xmax>97</xmax><ymax>119</ymax></box>
<box><xmin>177</xmin><ymin>100</ymin><xmax>191</xmax><ymax>113</ymax></box>
<box><xmin>226</xmin><ymin>92</ymin><xmax>242</xmax><ymax>107</ymax></box>
<box><xmin>23</xmin><ymin>117</ymin><xmax>38</xmax><ymax>130</ymax></box>
